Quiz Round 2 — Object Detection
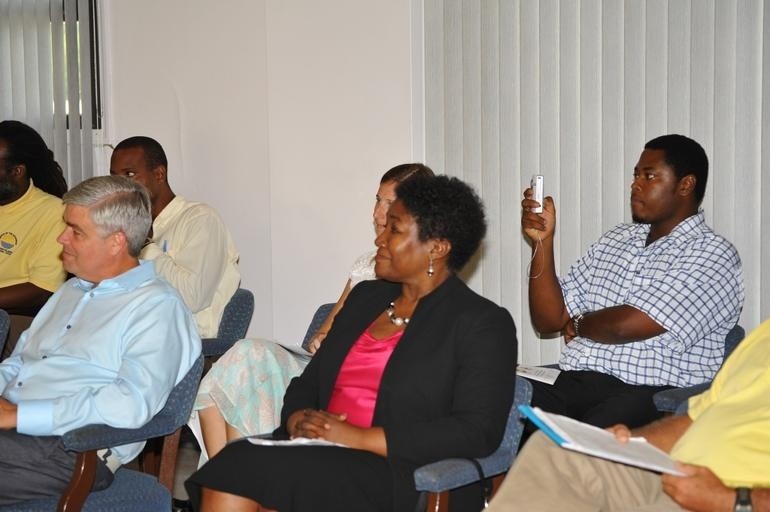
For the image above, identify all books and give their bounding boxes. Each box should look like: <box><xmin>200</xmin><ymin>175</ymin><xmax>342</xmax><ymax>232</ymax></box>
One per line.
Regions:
<box><xmin>243</xmin><ymin>435</ymin><xmax>350</xmax><ymax>448</ymax></box>
<box><xmin>516</xmin><ymin>364</ymin><xmax>562</xmax><ymax>387</ymax></box>
<box><xmin>516</xmin><ymin>402</ymin><xmax>688</xmax><ymax>476</ymax></box>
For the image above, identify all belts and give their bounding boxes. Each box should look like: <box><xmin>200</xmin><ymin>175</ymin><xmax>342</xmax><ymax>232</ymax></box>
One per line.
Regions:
<box><xmin>96</xmin><ymin>447</ymin><xmax>122</xmax><ymax>476</ymax></box>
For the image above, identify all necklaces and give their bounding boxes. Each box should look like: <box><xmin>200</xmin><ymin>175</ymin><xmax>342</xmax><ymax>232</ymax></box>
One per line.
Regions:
<box><xmin>384</xmin><ymin>300</ymin><xmax>411</xmax><ymax>327</ymax></box>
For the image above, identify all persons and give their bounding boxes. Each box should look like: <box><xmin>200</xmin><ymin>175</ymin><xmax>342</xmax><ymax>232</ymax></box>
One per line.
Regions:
<box><xmin>0</xmin><ymin>177</ymin><xmax>205</xmax><ymax>512</ymax></box>
<box><xmin>185</xmin><ymin>163</ymin><xmax>439</xmax><ymax>473</ymax></box>
<box><xmin>487</xmin><ymin>134</ymin><xmax>746</xmax><ymax>503</ymax></box>
<box><xmin>0</xmin><ymin>120</ymin><xmax>71</xmax><ymax>320</ymax></box>
<box><xmin>480</xmin><ymin>311</ymin><xmax>770</xmax><ymax>512</ymax></box>
<box><xmin>184</xmin><ymin>172</ymin><xmax>517</xmax><ymax>512</ymax></box>
<box><xmin>110</xmin><ymin>135</ymin><xmax>243</xmax><ymax>339</ymax></box>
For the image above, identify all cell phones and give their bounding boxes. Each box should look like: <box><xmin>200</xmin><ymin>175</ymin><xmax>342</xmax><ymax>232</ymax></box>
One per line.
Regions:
<box><xmin>530</xmin><ymin>175</ymin><xmax>543</xmax><ymax>213</ymax></box>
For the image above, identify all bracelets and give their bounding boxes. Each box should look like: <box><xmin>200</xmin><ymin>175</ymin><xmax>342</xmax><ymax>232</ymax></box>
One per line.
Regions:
<box><xmin>571</xmin><ymin>311</ymin><xmax>588</xmax><ymax>337</ymax></box>
<box><xmin>735</xmin><ymin>486</ymin><xmax>753</xmax><ymax>512</ymax></box>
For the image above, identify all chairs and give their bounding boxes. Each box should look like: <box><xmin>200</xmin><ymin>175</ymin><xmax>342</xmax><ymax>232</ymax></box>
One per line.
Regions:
<box><xmin>0</xmin><ymin>351</ymin><xmax>205</xmax><ymax>512</ymax></box>
<box><xmin>535</xmin><ymin>322</ymin><xmax>746</xmax><ymax>417</ymax></box>
<box><xmin>224</xmin><ymin>375</ymin><xmax>533</xmax><ymax>511</ymax></box>
<box><xmin>301</xmin><ymin>304</ymin><xmax>336</xmax><ymax>352</ymax></box>
<box><xmin>142</xmin><ymin>289</ymin><xmax>254</xmax><ymax>476</ymax></box>
<box><xmin>0</xmin><ymin>307</ymin><xmax>11</xmax><ymax>357</ymax></box>
